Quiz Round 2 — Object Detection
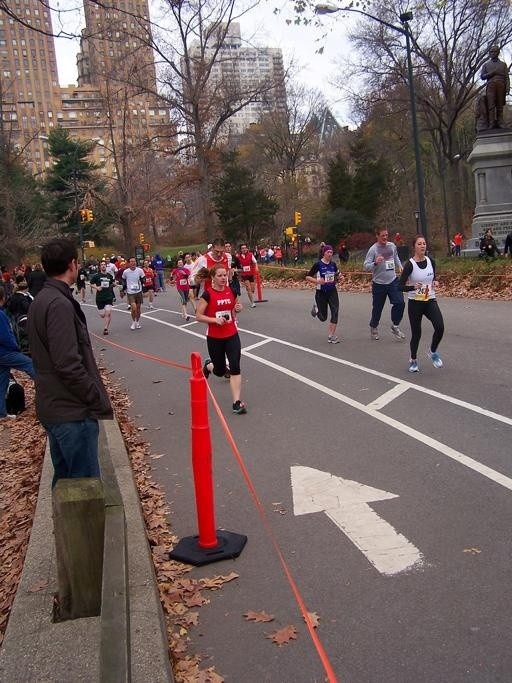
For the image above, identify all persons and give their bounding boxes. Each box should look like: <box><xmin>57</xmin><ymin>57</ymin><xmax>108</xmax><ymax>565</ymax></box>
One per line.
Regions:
<box><xmin>27</xmin><ymin>238</ymin><xmax>117</xmax><ymax>488</ymax></box>
<box><xmin>75</xmin><ymin>252</ymin><xmax>165</xmax><ymax>335</ymax></box>
<box><xmin>194</xmin><ymin>263</ymin><xmax>248</xmax><ymax>414</ymax></box>
<box><xmin>362</xmin><ymin>226</ymin><xmax>408</xmax><ymax>341</ymax></box>
<box><xmin>1</xmin><ymin>262</ymin><xmax>47</xmax><ymax>418</ymax></box>
<box><xmin>453</xmin><ymin>229</ymin><xmax>512</xmax><ymax>261</ymax></box>
<box><xmin>305</xmin><ymin>245</ymin><xmax>342</xmax><ymax>344</ymax></box>
<box><xmin>397</xmin><ymin>233</ymin><xmax>445</xmax><ymax>372</ymax></box>
<box><xmin>170</xmin><ymin>238</ymin><xmax>284</xmax><ymax>321</ymax></box>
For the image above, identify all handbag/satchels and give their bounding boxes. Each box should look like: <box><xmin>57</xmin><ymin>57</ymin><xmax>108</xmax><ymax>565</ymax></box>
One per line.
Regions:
<box><xmin>5</xmin><ymin>372</ymin><xmax>25</xmax><ymax>413</ymax></box>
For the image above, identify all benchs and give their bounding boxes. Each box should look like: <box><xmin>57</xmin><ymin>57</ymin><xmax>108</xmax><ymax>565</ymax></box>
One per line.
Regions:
<box><xmin>15</xmin><ymin>313</ymin><xmax>31</xmax><ymax>355</ymax></box>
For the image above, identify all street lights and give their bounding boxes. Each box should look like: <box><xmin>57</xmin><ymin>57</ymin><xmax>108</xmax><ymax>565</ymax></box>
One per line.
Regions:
<box><xmin>413</xmin><ymin>209</ymin><xmax>419</xmax><ymax>234</ymax></box>
<box><xmin>438</xmin><ymin>153</ymin><xmax>463</xmax><ymax>255</ymax></box>
<box><xmin>310</xmin><ymin>2</ymin><xmax>430</xmax><ymax>256</ymax></box>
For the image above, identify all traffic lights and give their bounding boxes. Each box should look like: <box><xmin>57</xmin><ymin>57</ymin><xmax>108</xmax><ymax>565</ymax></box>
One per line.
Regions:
<box><xmin>283</xmin><ymin>228</ymin><xmax>287</xmax><ymax>234</ymax></box>
<box><xmin>291</xmin><ymin>227</ymin><xmax>298</xmax><ymax>234</ymax></box>
<box><xmin>141</xmin><ymin>233</ymin><xmax>144</xmax><ymax>242</ymax></box>
<box><xmin>297</xmin><ymin>212</ymin><xmax>301</xmax><ymax>223</ymax></box>
<box><xmin>90</xmin><ymin>210</ymin><xmax>94</xmax><ymax>221</ymax></box>
<box><xmin>79</xmin><ymin>210</ymin><xmax>86</xmax><ymax>221</ymax></box>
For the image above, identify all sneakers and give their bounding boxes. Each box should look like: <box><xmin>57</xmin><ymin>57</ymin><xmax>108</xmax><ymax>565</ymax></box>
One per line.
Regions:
<box><xmin>391</xmin><ymin>324</ymin><xmax>406</xmax><ymax>339</ymax></box>
<box><xmin>371</xmin><ymin>326</ymin><xmax>380</xmax><ymax>340</ymax></box>
<box><xmin>328</xmin><ymin>334</ymin><xmax>340</xmax><ymax>343</ymax></box>
<box><xmin>186</xmin><ymin>315</ymin><xmax>190</xmax><ymax>322</ymax></box>
<box><xmin>130</xmin><ymin>322</ymin><xmax>135</xmax><ymax>329</ymax></box>
<box><xmin>409</xmin><ymin>358</ymin><xmax>418</xmax><ymax>372</ymax></box>
<box><xmin>233</xmin><ymin>399</ymin><xmax>247</xmax><ymax>412</ymax></box>
<box><xmin>135</xmin><ymin>323</ymin><xmax>140</xmax><ymax>328</ymax></box>
<box><xmin>427</xmin><ymin>348</ymin><xmax>443</xmax><ymax>368</ymax></box>
<box><xmin>311</xmin><ymin>303</ymin><xmax>317</xmax><ymax>319</ymax></box>
<box><xmin>0</xmin><ymin>414</ymin><xmax>16</xmax><ymax>421</ymax></box>
<box><xmin>104</xmin><ymin>328</ymin><xmax>108</xmax><ymax>334</ymax></box>
<box><xmin>203</xmin><ymin>358</ymin><xmax>212</xmax><ymax>378</ymax></box>
